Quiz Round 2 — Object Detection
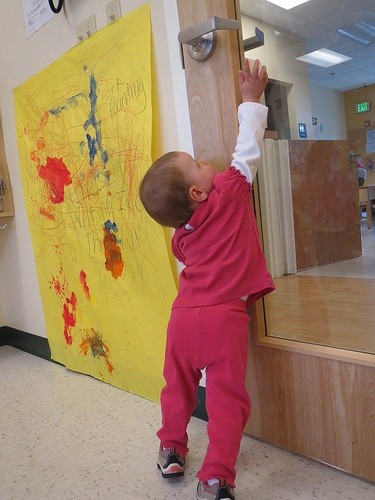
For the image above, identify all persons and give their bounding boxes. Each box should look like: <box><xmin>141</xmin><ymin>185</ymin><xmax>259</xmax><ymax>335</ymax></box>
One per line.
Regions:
<box><xmin>139</xmin><ymin>58</ymin><xmax>276</xmax><ymax>500</ymax></box>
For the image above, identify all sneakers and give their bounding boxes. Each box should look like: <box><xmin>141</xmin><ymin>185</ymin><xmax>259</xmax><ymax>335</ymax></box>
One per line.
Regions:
<box><xmin>196</xmin><ymin>480</ymin><xmax>235</xmax><ymax>500</ymax></box>
<box><xmin>157</xmin><ymin>446</ymin><xmax>185</xmax><ymax>478</ymax></box>
